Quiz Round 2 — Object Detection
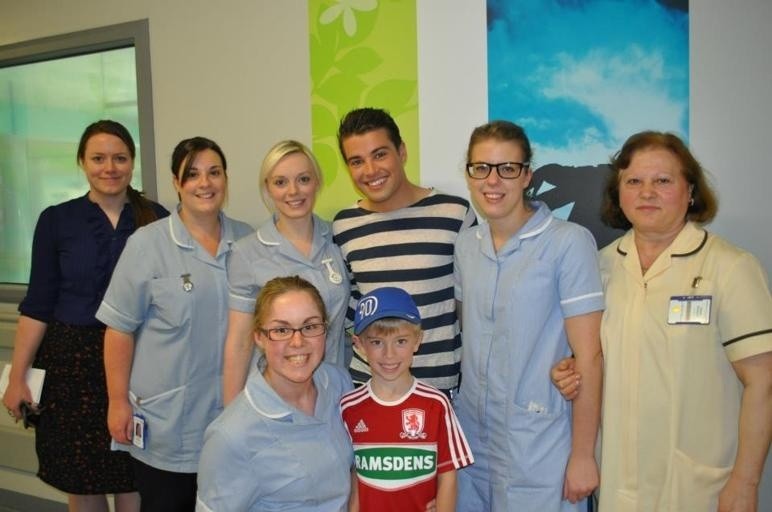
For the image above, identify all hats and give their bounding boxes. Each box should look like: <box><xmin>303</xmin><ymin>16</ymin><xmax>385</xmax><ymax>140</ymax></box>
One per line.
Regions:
<box><xmin>353</xmin><ymin>287</ymin><xmax>422</xmax><ymax>337</ymax></box>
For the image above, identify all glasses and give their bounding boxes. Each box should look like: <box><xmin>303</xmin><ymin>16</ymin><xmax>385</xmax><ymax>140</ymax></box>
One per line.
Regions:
<box><xmin>466</xmin><ymin>162</ymin><xmax>528</xmax><ymax>179</ymax></box>
<box><xmin>258</xmin><ymin>318</ymin><xmax>328</xmax><ymax>342</ymax></box>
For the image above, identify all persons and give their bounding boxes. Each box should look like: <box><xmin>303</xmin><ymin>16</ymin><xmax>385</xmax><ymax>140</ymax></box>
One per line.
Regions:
<box><xmin>0</xmin><ymin>118</ymin><xmax>171</xmax><ymax>510</ymax></box>
<box><xmin>190</xmin><ymin>274</ymin><xmax>354</xmax><ymax>512</ymax></box>
<box><xmin>448</xmin><ymin>119</ymin><xmax>606</xmax><ymax>512</ymax></box>
<box><xmin>327</xmin><ymin>107</ymin><xmax>474</xmax><ymax>420</ymax></box>
<box><xmin>94</xmin><ymin>136</ymin><xmax>256</xmax><ymax>511</ymax></box>
<box><xmin>550</xmin><ymin>130</ymin><xmax>771</xmax><ymax>511</ymax></box>
<box><xmin>219</xmin><ymin>138</ymin><xmax>354</xmax><ymax>410</ymax></box>
<box><xmin>337</xmin><ymin>286</ymin><xmax>475</xmax><ymax>510</ymax></box>
